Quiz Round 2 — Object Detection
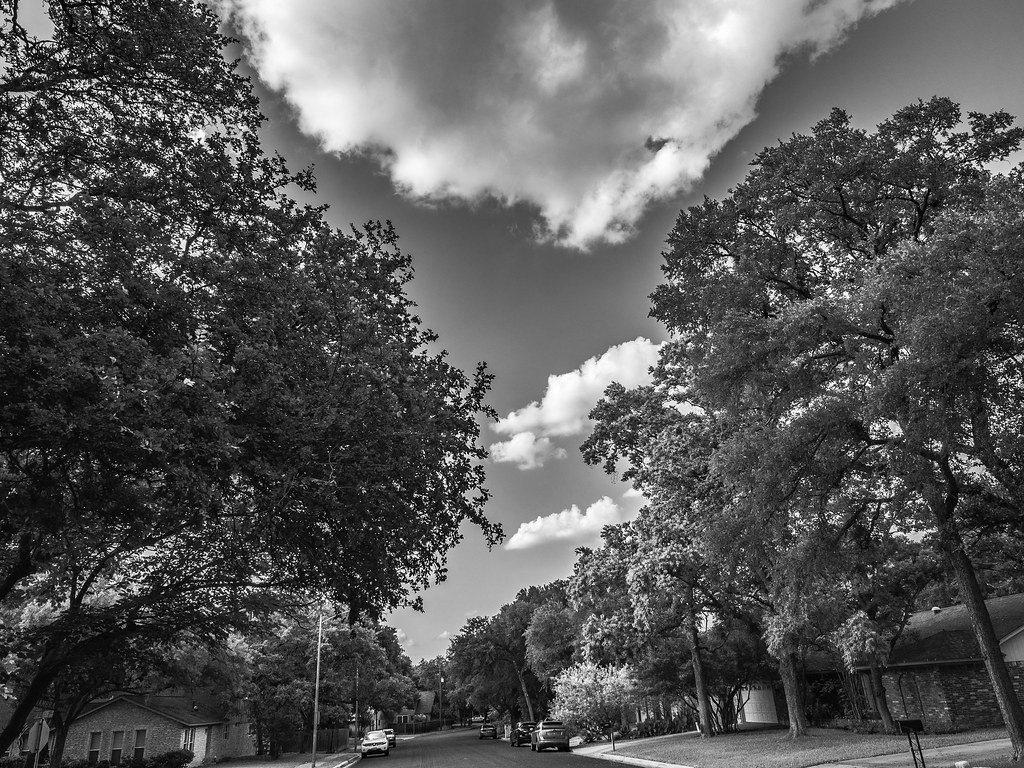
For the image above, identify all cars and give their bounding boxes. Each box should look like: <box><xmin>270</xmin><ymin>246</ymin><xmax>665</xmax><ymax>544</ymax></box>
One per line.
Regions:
<box><xmin>479</xmin><ymin>724</ymin><xmax>501</xmax><ymax>737</ymax></box>
<box><xmin>359</xmin><ymin>730</ymin><xmax>394</xmax><ymax>758</ymax></box>
<box><xmin>383</xmin><ymin>728</ymin><xmax>398</xmax><ymax>748</ymax></box>
<box><xmin>467</xmin><ymin>714</ymin><xmax>490</xmax><ymax>723</ymax></box>
<box><xmin>530</xmin><ymin>721</ymin><xmax>570</xmax><ymax>753</ymax></box>
<box><xmin>509</xmin><ymin>721</ymin><xmax>537</xmax><ymax>747</ymax></box>
